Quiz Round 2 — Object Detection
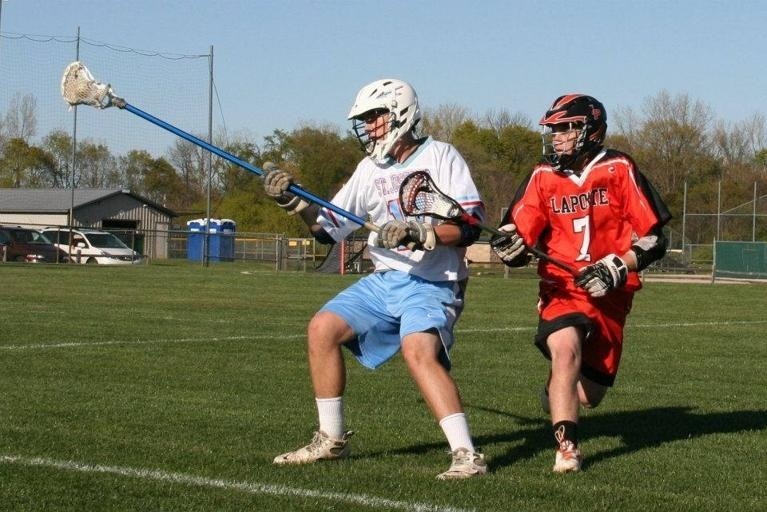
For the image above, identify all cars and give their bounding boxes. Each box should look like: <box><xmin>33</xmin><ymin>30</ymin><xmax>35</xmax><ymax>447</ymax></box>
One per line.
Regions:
<box><xmin>0</xmin><ymin>226</ymin><xmax>68</xmax><ymax>265</ymax></box>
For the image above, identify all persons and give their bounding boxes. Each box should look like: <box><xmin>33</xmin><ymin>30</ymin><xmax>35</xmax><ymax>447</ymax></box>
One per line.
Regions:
<box><xmin>262</xmin><ymin>77</ymin><xmax>492</xmax><ymax>483</ymax></box>
<box><xmin>488</xmin><ymin>91</ymin><xmax>676</xmax><ymax>476</ymax></box>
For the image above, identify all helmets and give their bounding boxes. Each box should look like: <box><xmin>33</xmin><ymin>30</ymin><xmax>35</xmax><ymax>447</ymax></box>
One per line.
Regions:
<box><xmin>347</xmin><ymin>78</ymin><xmax>422</xmax><ymax>164</ymax></box>
<box><xmin>537</xmin><ymin>93</ymin><xmax>607</xmax><ymax>174</ymax></box>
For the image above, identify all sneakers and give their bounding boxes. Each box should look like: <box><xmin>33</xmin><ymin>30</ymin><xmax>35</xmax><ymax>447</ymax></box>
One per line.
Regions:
<box><xmin>540</xmin><ymin>368</ymin><xmax>555</xmax><ymax>414</ymax></box>
<box><xmin>434</xmin><ymin>446</ymin><xmax>490</xmax><ymax>483</ymax></box>
<box><xmin>272</xmin><ymin>429</ymin><xmax>354</xmax><ymax>467</ymax></box>
<box><xmin>553</xmin><ymin>439</ymin><xmax>585</xmax><ymax>474</ymax></box>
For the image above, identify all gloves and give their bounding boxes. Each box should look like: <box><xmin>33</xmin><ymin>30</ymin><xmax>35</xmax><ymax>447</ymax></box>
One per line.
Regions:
<box><xmin>262</xmin><ymin>160</ymin><xmax>311</xmax><ymax>216</ymax></box>
<box><xmin>372</xmin><ymin>219</ymin><xmax>436</xmax><ymax>251</ymax></box>
<box><xmin>488</xmin><ymin>223</ymin><xmax>533</xmax><ymax>268</ymax></box>
<box><xmin>570</xmin><ymin>253</ymin><xmax>628</xmax><ymax>299</ymax></box>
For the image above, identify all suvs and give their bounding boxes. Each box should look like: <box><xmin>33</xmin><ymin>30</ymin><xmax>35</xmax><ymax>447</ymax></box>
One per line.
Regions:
<box><xmin>32</xmin><ymin>227</ymin><xmax>140</xmax><ymax>265</ymax></box>
